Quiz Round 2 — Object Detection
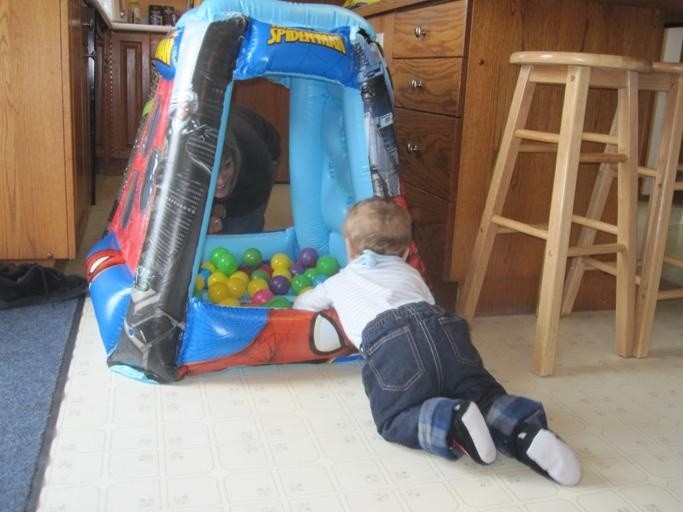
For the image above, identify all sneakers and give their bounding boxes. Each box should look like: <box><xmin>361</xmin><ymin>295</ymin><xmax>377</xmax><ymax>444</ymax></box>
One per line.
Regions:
<box><xmin>0</xmin><ymin>263</ymin><xmax>87</xmax><ymax>310</ymax></box>
<box><xmin>0</xmin><ymin>260</ymin><xmax>15</xmax><ymax>273</ymax></box>
<box><xmin>516</xmin><ymin>424</ymin><xmax>582</xmax><ymax>486</ymax></box>
<box><xmin>454</xmin><ymin>401</ymin><xmax>497</xmax><ymax>465</ymax></box>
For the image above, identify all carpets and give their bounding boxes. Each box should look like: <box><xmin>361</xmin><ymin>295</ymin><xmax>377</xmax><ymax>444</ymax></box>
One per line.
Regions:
<box><xmin>0</xmin><ymin>294</ymin><xmax>87</xmax><ymax>512</ymax></box>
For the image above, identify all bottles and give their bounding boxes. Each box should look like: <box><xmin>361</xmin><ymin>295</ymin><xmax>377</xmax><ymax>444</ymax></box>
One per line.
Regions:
<box><xmin>127</xmin><ymin>0</ymin><xmax>144</xmax><ymax>24</ymax></box>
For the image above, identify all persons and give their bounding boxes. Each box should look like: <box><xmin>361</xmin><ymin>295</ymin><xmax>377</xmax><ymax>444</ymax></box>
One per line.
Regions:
<box><xmin>207</xmin><ymin>106</ymin><xmax>280</xmax><ymax>234</ymax></box>
<box><xmin>291</xmin><ymin>196</ymin><xmax>584</xmax><ymax>489</ymax></box>
